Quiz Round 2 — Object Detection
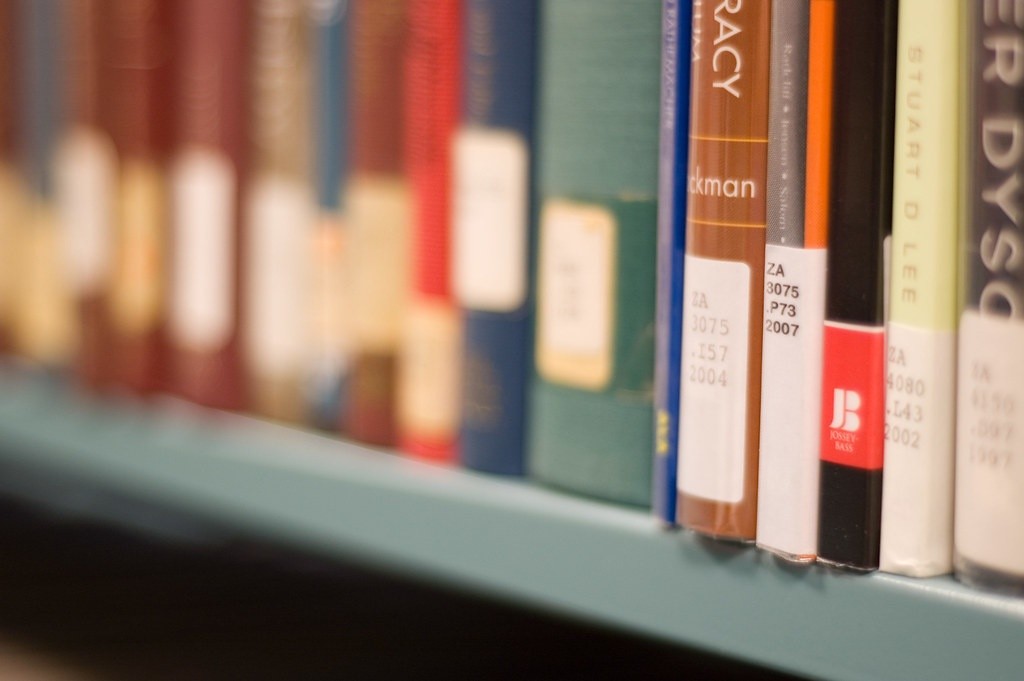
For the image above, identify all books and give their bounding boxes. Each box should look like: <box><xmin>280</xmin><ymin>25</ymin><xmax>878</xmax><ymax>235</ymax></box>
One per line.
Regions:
<box><xmin>1</xmin><ymin>0</ymin><xmax>1023</xmax><ymax>596</ymax></box>
